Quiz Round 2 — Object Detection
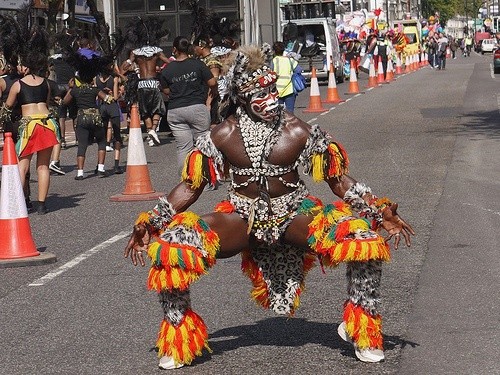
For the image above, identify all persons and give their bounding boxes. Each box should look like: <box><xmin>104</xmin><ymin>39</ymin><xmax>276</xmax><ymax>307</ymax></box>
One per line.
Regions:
<box><xmin>461</xmin><ymin>35</ymin><xmax>472</xmax><ymax>56</ymax></box>
<box><xmin>270</xmin><ymin>41</ymin><xmax>298</xmax><ymax>114</ymax></box>
<box><xmin>125</xmin><ymin>46</ymin><xmax>414</xmax><ymax>369</ymax></box>
<box><xmin>344</xmin><ymin>30</ymin><xmax>407</xmax><ymax>80</ymax></box>
<box><xmin>0</xmin><ymin>35</ymin><xmax>240</xmax><ymax>215</ymax></box>
<box><xmin>422</xmin><ymin>32</ymin><xmax>457</xmax><ymax>70</ymax></box>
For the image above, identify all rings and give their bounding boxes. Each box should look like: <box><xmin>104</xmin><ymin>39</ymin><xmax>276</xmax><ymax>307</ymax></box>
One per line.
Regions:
<box><xmin>138</xmin><ymin>251</ymin><xmax>141</xmax><ymax>254</ymax></box>
<box><xmin>396</xmin><ymin>234</ymin><xmax>399</xmax><ymax>235</ymax></box>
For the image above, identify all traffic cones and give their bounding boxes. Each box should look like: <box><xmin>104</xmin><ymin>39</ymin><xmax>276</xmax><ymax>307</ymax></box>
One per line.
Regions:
<box><xmin>386</xmin><ymin>51</ymin><xmax>430</xmax><ymax>89</ymax></box>
<box><xmin>302</xmin><ymin>67</ymin><xmax>330</xmax><ymax>112</ymax></box>
<box><xmin>0</xmin><ymin>131</ymin><xmax>58</xmax><ymax>269</ymax></box>
<box><xmin>108</xmin><ymin>104</ymin><xmax>168</xmax><ymax>201</ymax></box>
<box><xmin>322</xmin><ymin>62</ymin><xmax>346</xmax><ymax>104</ymax></box>
<box><xmin>343</xmin><ymin>59</ymin><xmax>366</xmax><ymax>95</ymax></box>
<box><xmin>375</xmin><ymin>55</ymin><xmax>391</xmax><ymax>85</ymax></box>
<box><xmin>364</xmin><ymin>55</ymin><xmax>382</xmax><ymax>88</ymax></box>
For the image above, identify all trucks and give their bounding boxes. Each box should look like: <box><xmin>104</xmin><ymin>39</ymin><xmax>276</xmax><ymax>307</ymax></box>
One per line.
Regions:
<box><xmin>393</xmin><ymin>19</ymin><xmax>423</xmax><ymax>56</ymax></box>
<box><xmin>274</xmin><ymin>16</ymin><xmax>345</xmax><ymax>85</ymax></box>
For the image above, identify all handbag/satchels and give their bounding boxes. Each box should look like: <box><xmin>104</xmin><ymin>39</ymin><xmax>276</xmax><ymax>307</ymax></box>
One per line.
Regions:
<box><xmin>292</xmin><ymin>73</ymin><xmax>307</xmax><ymax>96</ymax></box>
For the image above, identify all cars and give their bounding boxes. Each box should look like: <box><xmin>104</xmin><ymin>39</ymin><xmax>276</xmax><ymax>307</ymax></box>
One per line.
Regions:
<box><xmin>494</xmin><ymin>46</ymin><xmax>500</xmax><ymax>74</ymax></box>
<box><xmin>473</xmin><ymin>32</ymin><xmax>498</xmax><ymax>55</ymax></box>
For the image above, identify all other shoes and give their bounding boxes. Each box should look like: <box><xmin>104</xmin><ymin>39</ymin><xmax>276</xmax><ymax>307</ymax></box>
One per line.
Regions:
<box><xmin>95</xmin><ymin>168</ymin><xmax>98</xmax><ymax>175</ymax></box>
<box><xmin>148</xmin><ymin>140</ymin><xmax>154</xmax><ymax>148</ymax></box>
<box><xmin>74</xmin><ymin>175</ymin><xmax>83</xmax><ymax>180</ymax></box>
<box><xmin>75</xmin><ymin>142</ymin><xmax>79</xmax><ymax>147</ymax></box>
<box><xmin>49</xmin><ymin>162</ymin><xmax>65</xmax><ymax>175</ymax></box>
<box><xmin>60</xmin><ymin>144</ymin><xmax>67</xmax><ymax>150</ymax></box>
<box><xmin>147</xmin><ymin>129</ymin><xmax>161</xmax><ymax>145</ymax></box>
<box><xmin>97</xmin><ymin>171</ymin><xmax>108</xmax><ymax>177</ymax></box>
<box><xmin>106</xmin><ymin>146</ymin><xmax>113</xmax><ymax>152</ymax></box>
<box><xmin>26</xmin><ymin>204</ymin><xmax>33</xmax><ymax>214</ymax></box>
<box><xmin>113</xmin><ymin>166</ymin><xmax>124</xmax><ymax>175</ymax></box>
<box><xmin>37</xmin><ymin>206</ymin><xmax>49</xmax><ymax>215</ymax></box>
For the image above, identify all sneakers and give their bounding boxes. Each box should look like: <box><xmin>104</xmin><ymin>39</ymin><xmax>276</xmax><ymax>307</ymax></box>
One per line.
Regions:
<box><xmin>159</xmin><ymin>351</ymin><xmax>185</xmax><ymax>370</ymax></box>
<box><xmin>337</xmin><ymin>319</ymin><xmax>386</xmax><ymax>364</ymax></box>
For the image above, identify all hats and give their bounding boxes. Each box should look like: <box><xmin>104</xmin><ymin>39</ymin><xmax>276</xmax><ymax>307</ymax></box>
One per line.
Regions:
<box><xmin>218</xmin><ymin>42</ymin><xmax>279</xmax><ymax>98</ymax></box>
